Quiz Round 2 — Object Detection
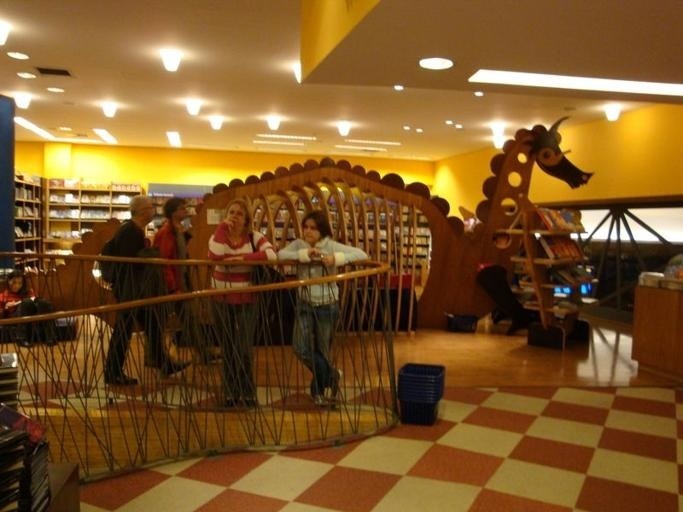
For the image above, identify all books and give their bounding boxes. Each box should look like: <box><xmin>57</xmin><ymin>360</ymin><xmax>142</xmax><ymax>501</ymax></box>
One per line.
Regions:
<box><xmin>0</xmin><ymin>352</ymin><xmax>51</xmax><ymax>512</ymax></box>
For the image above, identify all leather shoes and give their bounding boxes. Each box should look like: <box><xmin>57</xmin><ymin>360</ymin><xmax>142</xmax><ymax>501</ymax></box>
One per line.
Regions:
<box><xmin>105</xmin><ymin>370</ymin><xmax>136</xmax><ymax>385</ymax></box>
<box><xmin>45</xmin><ymin>339</ymin><xmax>54</xmax><ymax>346</ymax></box>
<box><xmin>159</xmin><ymin>359</ymin><xmax>192</xmax><ymax>377</ymax></box>
<box><xmin>17</xmin><ymin>339</ymin><xmax>32</xmax><ymax>348</ymax></box>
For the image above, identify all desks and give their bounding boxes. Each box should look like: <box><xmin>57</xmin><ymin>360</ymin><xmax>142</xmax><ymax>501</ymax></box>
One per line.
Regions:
<box><xmin>630</xmin><ymin>283</ymin><xmax>681</xmax><ymax>388</ymax></box>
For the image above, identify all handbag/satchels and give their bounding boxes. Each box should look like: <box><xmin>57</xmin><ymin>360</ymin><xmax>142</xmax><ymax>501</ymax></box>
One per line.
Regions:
<box><xmin>257</xmin><ymin>265</ymin><xmax>293</xmax><ymax>315</ymax></box>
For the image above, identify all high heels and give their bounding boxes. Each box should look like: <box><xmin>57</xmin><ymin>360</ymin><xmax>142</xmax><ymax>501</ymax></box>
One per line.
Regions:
<box><xmin>223</xmin><ymin>396</ymin><xmax>257</xmax><ymax>408</ymax></box>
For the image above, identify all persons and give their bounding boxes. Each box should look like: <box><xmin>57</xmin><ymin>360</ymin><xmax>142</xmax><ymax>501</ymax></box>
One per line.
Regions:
<box><xmin>0</xmin><ymin>271</ymin><xmax>56</xmax><ymax>347</ymax></box>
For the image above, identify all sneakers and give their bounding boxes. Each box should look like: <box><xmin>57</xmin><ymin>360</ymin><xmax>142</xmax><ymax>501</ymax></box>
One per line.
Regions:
<box><xmin>306</xmin><ymin>370</ymin><xmax>343</xmax><ymax>406</ymax></box>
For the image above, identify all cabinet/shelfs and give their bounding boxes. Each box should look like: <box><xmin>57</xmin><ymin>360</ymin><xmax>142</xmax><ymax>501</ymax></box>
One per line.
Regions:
<box><xmin>257</xmin><ymin>219</ymin><xmax>428</xmax><ymax>275</ymax></box>
<box><xmin>10</xmin><ymin>174</ymin><xmax>145</xmax><ymax>272</ymax></box>
<box><xmin>512</xmin><ymin>210</ymin><xmax>586</xmax><ymax>329</ymax></box>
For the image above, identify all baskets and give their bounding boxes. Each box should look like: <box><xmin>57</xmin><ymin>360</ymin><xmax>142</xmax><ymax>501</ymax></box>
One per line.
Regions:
<box><xmin>399</xmin><ymin>358</ymin><xmax>446</xmax><ymax>424</ymax></box>
